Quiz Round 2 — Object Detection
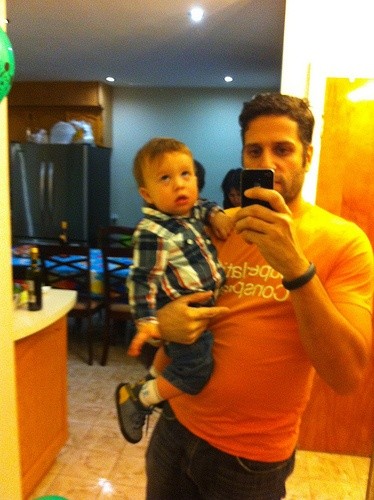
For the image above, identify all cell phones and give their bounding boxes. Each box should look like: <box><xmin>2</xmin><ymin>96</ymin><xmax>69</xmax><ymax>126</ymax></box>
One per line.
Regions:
<box><xmin>240</xmin><ymin>167</ymin><xmax>276</xmax><ymax>213</ymax></box>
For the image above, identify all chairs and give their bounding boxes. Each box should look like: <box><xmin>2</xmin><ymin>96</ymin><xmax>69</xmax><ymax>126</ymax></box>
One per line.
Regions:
<box><xmin>37</xmin><ymin>245</ymin><xmax>102</xmax><ymax>366</ymax></box>
<box><xmin>100</xmin><ymin>224</ymin><xmax>135</xmax><ymax>247</ymax></box>
<box><xmin>101</xmin><ymin>246</ymin><xmax>153</xmax><ymax>369</ymax></box>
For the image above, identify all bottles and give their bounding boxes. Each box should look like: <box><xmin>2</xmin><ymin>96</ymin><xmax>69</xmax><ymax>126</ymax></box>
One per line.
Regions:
<box><xmin>26</xmin><ymin>247</ymin><xmax>43</xmax><ymax>311</ymax></box>
<box><xmin>58</xmin><ymin>220</ymin><xmax>70</xmax><ymax>258</ymax></box>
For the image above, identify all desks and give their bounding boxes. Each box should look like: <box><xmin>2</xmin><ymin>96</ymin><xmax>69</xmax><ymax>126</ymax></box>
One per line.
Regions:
<box><xmin>12</xmin><ymin>244</ymin><xmax>133</xmax><ymax>327</ymax></box>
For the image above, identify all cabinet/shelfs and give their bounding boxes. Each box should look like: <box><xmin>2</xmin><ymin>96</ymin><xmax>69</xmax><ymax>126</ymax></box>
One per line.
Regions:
<box><xmin>14</xmin><ymin>279</ymin><xmax>77</xmax><ymax>500</ymax></box>
<box><xmin>8</xmin><ymin>79</ymin><xmax>114</xmax><ymax>143</ymax></box>
<box><xmin>11</xmin><ymin>146</ymin><xmax>110</xmax><ymax>245</ymax></box>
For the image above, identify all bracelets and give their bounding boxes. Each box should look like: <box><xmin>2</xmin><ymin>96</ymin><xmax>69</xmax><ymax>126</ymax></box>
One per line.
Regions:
<box><xmin>282</xmin><ymin>262</ymin><xmax>317</xmax><ymax>290</ymax></box>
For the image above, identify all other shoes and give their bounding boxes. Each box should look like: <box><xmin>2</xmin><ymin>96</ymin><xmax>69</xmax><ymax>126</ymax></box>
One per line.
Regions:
<box><xmin>115</xmin><ymin>380</ymin><xmax>154</xmax><ymax>444</ymax></box>
<box><xmin>133</xmin><ymin>373</ymin><xmax>176</xmax><ymax>421</ymax></box>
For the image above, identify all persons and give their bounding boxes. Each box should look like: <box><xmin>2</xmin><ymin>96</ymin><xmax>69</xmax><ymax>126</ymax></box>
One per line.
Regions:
<box><xmin>115</xmin><ymin>139</ymin><xmax>236</xmax><ymax>444</ymax></box>
<box><xmin>144</xmin><ymin>94</ymin><xmax>374</xmax><ymax>500</ymax></box>
<box><xmin>194</xmin><ymin>159</ymin><xmax>244</xmax><ymax>209</ymax></box>
